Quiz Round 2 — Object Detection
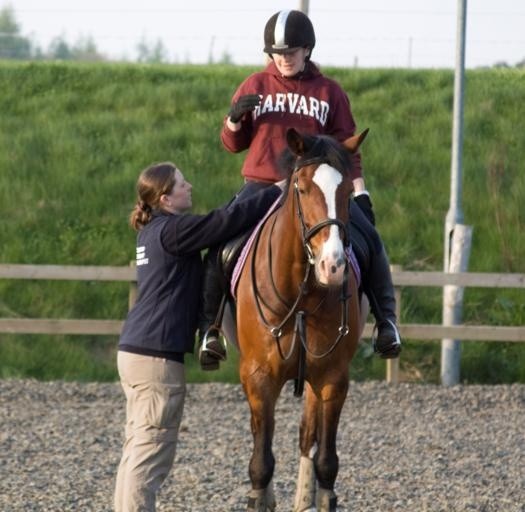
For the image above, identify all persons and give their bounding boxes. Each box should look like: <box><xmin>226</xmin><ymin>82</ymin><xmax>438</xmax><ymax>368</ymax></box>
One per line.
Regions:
<box><xmin>197</xmin><ymin>11</ymin><xmax>401</xmax><ymax>369</ymax></box>
<box><xmin>115</xmin><ymin>165</ymin><xmax>287</xmax><ymax>512</ymax></box>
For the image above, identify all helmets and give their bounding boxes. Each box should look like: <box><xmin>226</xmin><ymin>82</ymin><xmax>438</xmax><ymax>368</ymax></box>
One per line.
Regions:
<box><xmin>264</xmin><ymin>10</ymin><xmax>315</xmax><ymax>53</ymax></box>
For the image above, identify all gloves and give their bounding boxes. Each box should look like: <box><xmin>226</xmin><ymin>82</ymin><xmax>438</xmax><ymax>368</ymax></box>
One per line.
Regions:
<box><xmin>230</xmin><ymin>95</ymin><xmax>261</xmax><ymax>123</ymax></box>
<box><xmin>353</xmin><ymin>191</ymin><xmax>375</xmax><ymax>225</ymax></box>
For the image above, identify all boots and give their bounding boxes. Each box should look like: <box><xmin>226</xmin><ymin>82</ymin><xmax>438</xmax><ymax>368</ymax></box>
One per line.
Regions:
<box><xmin>366</xmin><ymin>247</ymin><xmax>400</xmax><ymax>357</ymax></box>
<box><xmin>198</xmin><ymin>253</ymin><xmax>226</xmax><ymax>369</ymax></box>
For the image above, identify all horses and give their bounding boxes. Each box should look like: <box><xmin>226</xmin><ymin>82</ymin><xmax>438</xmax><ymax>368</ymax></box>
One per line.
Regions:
<box><xmin>222</xmin><ymin>123</ymin><xmax>369</xmax><ymax>512</ymax></box>
